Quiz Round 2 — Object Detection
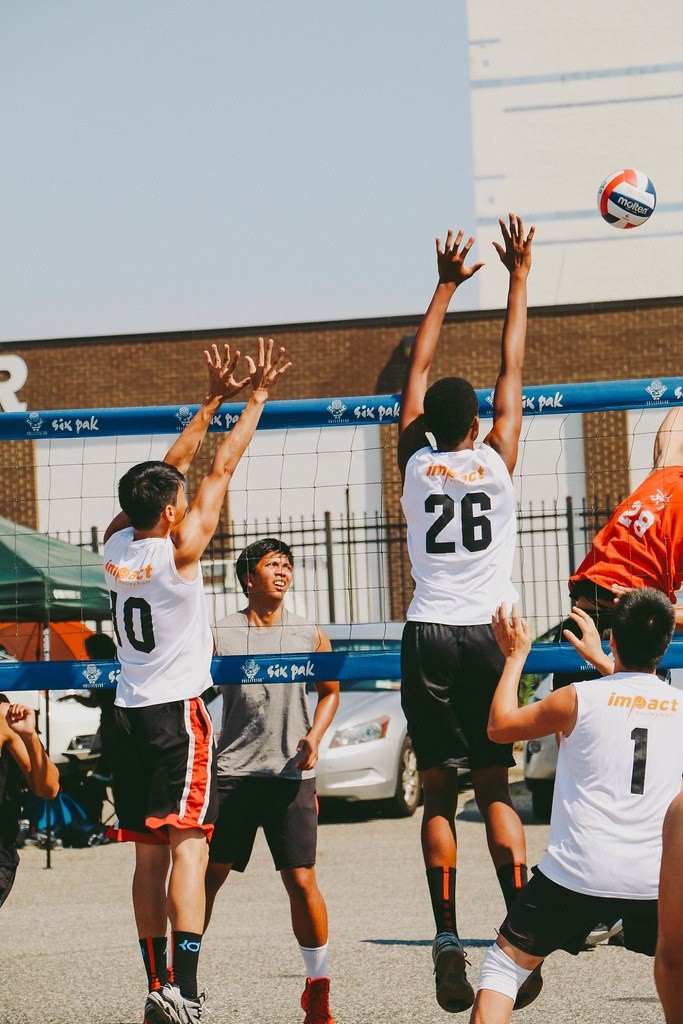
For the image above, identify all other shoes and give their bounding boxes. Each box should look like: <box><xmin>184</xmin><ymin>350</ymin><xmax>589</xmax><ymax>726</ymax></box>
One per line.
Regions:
<box><xmin>512</xmin><ymin>956</ymin><xmax>543</xmax><ymax>1010</ymax></box>
<box><xmin>584</xmin><ymin>918</ymin><xmax>625</xmax><ymax>945</ymax></box>
<box><xmin>432</xmin><ymin>932</ymin><xmax>476</xmax><ymax>1013</ymax></box>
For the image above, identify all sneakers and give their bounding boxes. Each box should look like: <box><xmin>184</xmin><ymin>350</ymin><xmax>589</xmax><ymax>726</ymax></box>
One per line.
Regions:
<box><xmin>143</xmin><ymin>969</ymin><xmax>209</xmax><ymax>1024</ymax></box>
<box><xmin>300</xmin><ymin>976</ymin><xmax>335</xmax><ymax>1024</ymax></box>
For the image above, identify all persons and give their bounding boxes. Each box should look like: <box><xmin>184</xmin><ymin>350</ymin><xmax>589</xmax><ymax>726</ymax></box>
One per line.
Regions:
<box><xmin>398</xmin><ymin>213</ymin><xmax>543</xmax><ymax>1013</ymax></box>
<box><xmin>102</xmin><ymin>338</ymin><xmax>293</xmax><ymax>1024</ymax></box>
<box><xmin>552</xmin><ymin>406</ymin><xmax>683</xmax><ymax>751</ymax></box>
<box><xmin>205</xmin><ymin>539</ymin><xmax>339</xmax><ymax>1024</ymax></box>
<box><xmin>0</xmin><ymin>692</ymin><xmax>60</xmax><ymax>906</ymax></box>
<box><xmin>57</xmin><ymin>633</ymin><xmax>116</xmax><ymax>747</ymax></box>
<box><xmin>467</xmin><ymin>587</ymin><xmax>683</xmax><ymax>1024</ymax></box>
<box><xmin>653</xmin><ymin>790</ymin><xmax>683</xmax><ymax>1024</ymax></box>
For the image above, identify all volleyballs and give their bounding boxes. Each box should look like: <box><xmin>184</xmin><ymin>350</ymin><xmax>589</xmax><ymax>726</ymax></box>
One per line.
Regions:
<box><xmin>595</xmin><ymin>166</ymin><xmax>658</xmax><ymax>231</ymax></box>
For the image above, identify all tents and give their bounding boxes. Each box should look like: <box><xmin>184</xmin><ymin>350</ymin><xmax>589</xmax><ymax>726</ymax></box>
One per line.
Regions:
<box><xmin>0</xmin><ymin>515</ymin><xmax>112</xmax><ymax>661</ymax></box>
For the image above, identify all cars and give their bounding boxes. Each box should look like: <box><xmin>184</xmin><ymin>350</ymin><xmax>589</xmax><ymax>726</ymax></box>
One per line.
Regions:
<box><xmin>0</xmin><ymin>651</ymin><xmax>101</xmax><ymax>769</ymax></box>
<box><xmin>204</xmin><ymin>624</ymin><xmax>471</xmax><ymax>818</ymax></box>
<box><xmin>523</xmin><ymin>667</ymin><xmax>683</xmax><ymax>822</ymax></box>
